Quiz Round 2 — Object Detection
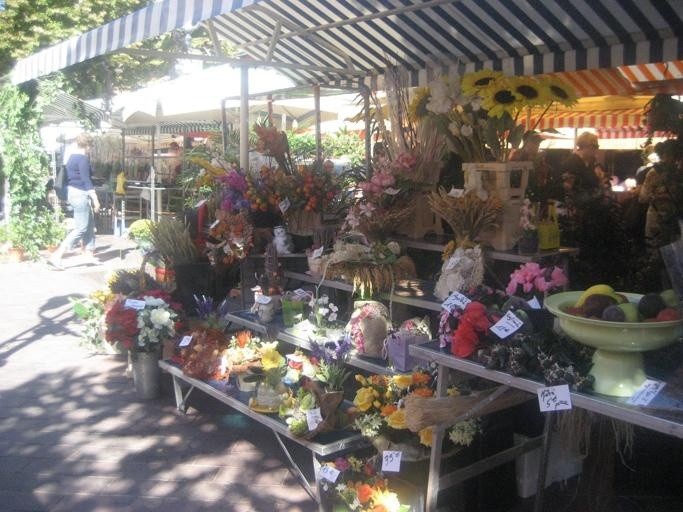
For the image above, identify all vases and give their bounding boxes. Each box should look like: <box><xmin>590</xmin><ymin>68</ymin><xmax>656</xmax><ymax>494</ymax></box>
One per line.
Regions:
<box><xmin>523</xmin><ymin>294</ymin><xmax>551</xmax><ymax>336</ymax></box>
<box><xmin>360</xmin><ymin>416</ymin><xmax>456</xmax><ymax>460</ymax></box>
<box><xmin>394</xmin><ymin>185</ymin><xmax>443</xmax><ymax>244</ymax></box>
<box><xmin>127</xmin><ymin>348</ymin><xmax>160</xmax><ymax>404</ymax></box>
<box><xmin>255</xmin><ymin>384</ymin><xmax>284</xmax><ymax>404</ymax></box>
<box><xmin>154</xmin><ymin>267</ymin><xmax>176</xmax><ymax>289</ymax></box>
<box><xmin>463</xmin><ymin>157</ymin><xmax>532</xmax><ymax>205</ymax></box>
<box><xmin>311</xmin><ymin>381</ymin><xmax>343</xmax><ymax>430</ymax></box>
<box><xmin>284</xmin><ymin>207</ymin><xmax>323</xmax><ymax>238</ymax></box>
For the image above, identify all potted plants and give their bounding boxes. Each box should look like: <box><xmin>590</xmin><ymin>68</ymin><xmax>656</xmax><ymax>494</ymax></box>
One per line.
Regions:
<box><xmin>7</xmin><ymin>209</ymin><xmax>27</xmax><ymax>263</ymax></box>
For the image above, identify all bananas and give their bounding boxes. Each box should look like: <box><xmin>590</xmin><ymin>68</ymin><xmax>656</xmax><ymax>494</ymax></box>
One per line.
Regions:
<box><xmin>575</xmin><ymin>284</ymin><xmax>622</xmax><ymax>309</ymax></box>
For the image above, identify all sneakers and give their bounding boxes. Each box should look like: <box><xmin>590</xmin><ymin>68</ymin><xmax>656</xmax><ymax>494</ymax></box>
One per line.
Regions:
<box><xmin>82</xmin><ymin>256</ymin><xmax>103</xmax><ymax>265</ymax></box>
<box><xmin>46</xmin><ymin>259</ymin><xmax>64</xmax><ymax>270</ymax></box>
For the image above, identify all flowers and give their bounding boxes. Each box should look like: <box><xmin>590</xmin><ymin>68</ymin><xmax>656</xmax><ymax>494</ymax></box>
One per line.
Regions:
<box><xmin>222</xmin><ymin>327</ymin><xmax>280</xmax><ymax>375</ymax></box>
<box><xmin>508</xmin><ymin>258</ymin><xmax>567</xmax><ymax>295</ymax></box>
<box><xmin>259</xmin><ymin>350</ymin><xmax>283</xmax><ymax>388</ymax></box>
<box><xmin>408</xmin><ymin>64</ymin><xmax>580</xmax><ymax>163</ymax></box>
<box><xmin>352</xmin><ymin>373</ymin><xmax>484</xmax><ymax>448</ymax></box>
<box><xmin>252</xmin><ymin>120</ymin><xmax>352</xmax><ymax>215</ymax></box>
<box><xmin>314</xmin><ymin>333</ymin><xmax>346</xmax><ymax>386</ymax></box>
<box><xmin>102</xmin><ymin>289</ymin><xmax>181</xmax><ymax>358</ymax></box>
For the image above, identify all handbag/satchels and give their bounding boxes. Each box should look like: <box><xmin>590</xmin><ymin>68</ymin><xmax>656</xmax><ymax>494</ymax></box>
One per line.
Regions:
<box><xmin>54</xmin><ymin>165</ymin><xmax>67</xmax><ymax>200</ymax></box>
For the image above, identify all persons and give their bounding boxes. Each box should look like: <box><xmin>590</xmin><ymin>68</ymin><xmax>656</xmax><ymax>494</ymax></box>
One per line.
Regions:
<box><xmin>170</xmin><ymin>141</ymin><xmax>182</xmax><ymax>174</ymax></box>
<box><xmin>47</xmin><ymin>133</ymin><xmax>100</xmax><ymax>270</ymax></box>
<box><xmin>638</xmin><ymin>137</ymin><xmax>682</xmax><ymax>260</ymax></box>
<box><xmin>596</xmin><ymin>152</ymin><xmax>660</xmax><ymax>191</ymax></box>
<box><xmin>561</xmin><ymin>130</ymin><xmax>597</xmax><ymax>194</ymax></box>
<box><xmin>511</xmin><ymin>130</ymin><xmax>544</xmax><ymax>162</ymax></box>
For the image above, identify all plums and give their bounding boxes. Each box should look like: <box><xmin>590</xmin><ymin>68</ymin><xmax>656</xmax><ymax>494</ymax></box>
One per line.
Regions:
<box><xmin>582</xmin><ymin>290</ymin><xmax>682</xmax><ymax>322</ymax></box>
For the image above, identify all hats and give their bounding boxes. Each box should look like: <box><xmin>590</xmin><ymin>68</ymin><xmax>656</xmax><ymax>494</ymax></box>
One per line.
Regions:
<box><xmin>523</xmin><ymin>131</ymin><xmax>544</xmax><ymax>142</ymax></box>
